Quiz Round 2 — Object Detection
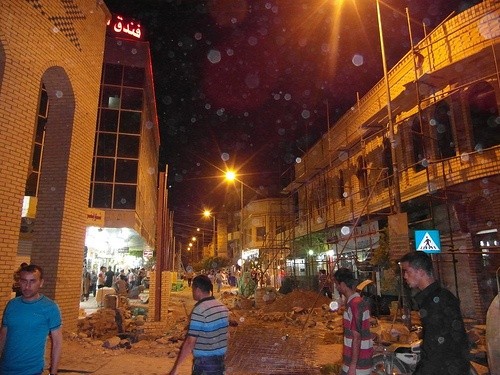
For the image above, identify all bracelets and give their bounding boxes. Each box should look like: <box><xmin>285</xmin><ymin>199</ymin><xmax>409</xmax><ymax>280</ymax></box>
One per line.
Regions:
<box><xmin>50</xmin><ymin>374</ymin><xmax>57</xmax><ymax>375</ymax></box>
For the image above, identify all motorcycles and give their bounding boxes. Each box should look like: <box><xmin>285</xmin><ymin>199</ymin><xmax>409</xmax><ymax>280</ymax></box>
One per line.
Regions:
<box><xmin>370</xmin><ymin>328</ymin><xmax>480</xmax><ymax>375</ymax></box>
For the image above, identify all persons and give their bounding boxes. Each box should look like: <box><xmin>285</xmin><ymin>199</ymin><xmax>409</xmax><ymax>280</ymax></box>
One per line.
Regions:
<box><xmin>399</xmin><ymin>251</ymin><xmax>472</xmax><ymax>375</ymax></box>
<box><xmin>80</xmin><ymin>266</ymin><xmax>150</xmax><ymax>303</ymax></box>
<box><xmin>181</xmin><ymin>267</ymin><xmax>238</xmax><ymax>292</ymax></box>
<box><xmin>320</xmin><ymin>269</ymin><xmax>332</xmax><ymax>299</ymax></box>
<box><xmin>0</xmin><ymin>264</ymin><xmax>64</xmax><ymax>375</ymax></box>
<box><xmin>334</xmin><ymin>267</ymin><xmax>376</xmax><ymax>374</ymax></box>
<box><xmin>485</xmin><ymin>267</ymin><xmax>500</xmax><ymax>374</ymax></box>
<box><xmin>168</xmin><ymin>274</ymin><xmax>230</xmax><ymax>375</ymax></box>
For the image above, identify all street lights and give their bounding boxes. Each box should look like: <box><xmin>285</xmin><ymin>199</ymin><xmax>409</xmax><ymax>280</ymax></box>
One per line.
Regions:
<box><xmin>204</xmin><ymin>209</ymin><xmax>219</xmax><ymax>260</ymax></box>
<box><xmin>223</xmin><ymin>168</ymin><xmax>245</xmax><ymax>248</ymax></box>
<box><xmin>187</xmin><ymin>226</ymin><xmax>205</xmax><ymax>265</ymax></box>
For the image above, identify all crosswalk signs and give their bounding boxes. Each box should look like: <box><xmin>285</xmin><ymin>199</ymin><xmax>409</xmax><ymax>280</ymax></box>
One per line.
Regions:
<box><xmin>415</xmin><ymin>231</ymin><xmax>441</xmax><ymax>253</ymax></box>
